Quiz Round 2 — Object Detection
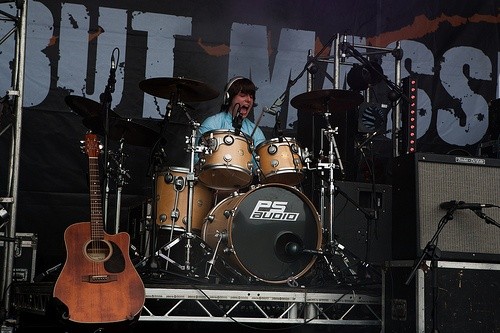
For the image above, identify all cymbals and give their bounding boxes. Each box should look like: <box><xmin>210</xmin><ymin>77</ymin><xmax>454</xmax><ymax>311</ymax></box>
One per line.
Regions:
<box><xmin>139</xmin><ymin>77</ymin><xmax>220</xmax><ymax>102</ymax></box>
<box><xmin>64</xmin><ymin>95</ymin><xmax>121</xmax><ymax>117</ymax></box>
<box><xmin>82</xmin><ymin>117</ymin><xmax>160</xmax><ymax>141</ymax></box>
<box><xmin>290</xmin><ymin>89</ymin><xmax>365</xmax><ymax>112</ymax></box>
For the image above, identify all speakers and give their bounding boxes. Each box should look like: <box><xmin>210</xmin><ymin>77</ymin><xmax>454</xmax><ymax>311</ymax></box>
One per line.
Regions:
<box><xmin>385</xmin><ymin>153</ymin><xmax>500</xmax><ymax>263</ymax></box>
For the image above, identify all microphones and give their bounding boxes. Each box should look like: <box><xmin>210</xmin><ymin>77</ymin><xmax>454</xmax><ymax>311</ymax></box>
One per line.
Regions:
<box><xmin>439</xmin><ymin>201</ymin><xmax>492</xmax><ymax>208</ymax></box>
<box><xmin>283</xmin><ymin>243</ymin><xmax>302</xmax><ymax>259</ymax></box>
<box><xmin>110</xmin><ymin>56</ymin><xmax>116</xmax><ymax>92</ymax></box>
<box><xmin>263</xmin><ymin>106</ymin><xmax>279</xmax><ymax>116</ymax></box>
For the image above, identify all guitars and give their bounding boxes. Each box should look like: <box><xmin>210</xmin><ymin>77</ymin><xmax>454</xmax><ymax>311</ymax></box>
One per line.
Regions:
<box><xmin>52</xmin><ymin>130</ymin><xmax>146</xmax><ymax>323</ymax></box>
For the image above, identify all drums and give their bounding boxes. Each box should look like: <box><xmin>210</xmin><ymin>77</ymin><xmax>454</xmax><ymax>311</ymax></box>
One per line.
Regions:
<box><xmin>155</xmin><ymin>166</ymin><xmax>216</xmax><ymax>234</ymax></box>
<box><xmin>256</xmin><ymin>137</ymin><xmax>306</xmax><ymax>185</ymax></box>
<box><xmin>196</xmin><ymin>129</ymin><xmax>254</xmax><ymax>190</ymax></box>
<box><xmin>201</xmin><ymin>183</ymin><xmax>322</xmax><ymax>283</ymax></box>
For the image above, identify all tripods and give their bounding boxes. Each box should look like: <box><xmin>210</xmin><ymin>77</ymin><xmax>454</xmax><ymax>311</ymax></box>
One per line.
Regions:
<box><xmin>127</xmin><ymin>102</ymin><xmax>372</xmax><ymax>287</ymax></box>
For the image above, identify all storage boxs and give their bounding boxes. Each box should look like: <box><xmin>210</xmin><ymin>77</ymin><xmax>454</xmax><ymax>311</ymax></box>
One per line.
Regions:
<box><xmin>382</xmin><ymin>260</ymin><xmax>500</xmax><ymax>333</ymax></box>
<box><xmin>0</xmin><ymin>232</ymin><xmax>37</xmax><ymax>310</ymax></box>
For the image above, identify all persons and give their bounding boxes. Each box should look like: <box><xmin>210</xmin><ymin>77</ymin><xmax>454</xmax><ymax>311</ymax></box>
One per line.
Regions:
<box><xmin>196</xmin><ymin>78</ymin><xmax>267</xmax><ymax>190</ymax></box>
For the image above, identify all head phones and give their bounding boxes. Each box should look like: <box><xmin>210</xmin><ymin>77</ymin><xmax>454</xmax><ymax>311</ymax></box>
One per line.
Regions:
<box><xmin>222</xmin><ymin>76</ymin><xmax>245</xmax><ymax>104</ymax></box>
